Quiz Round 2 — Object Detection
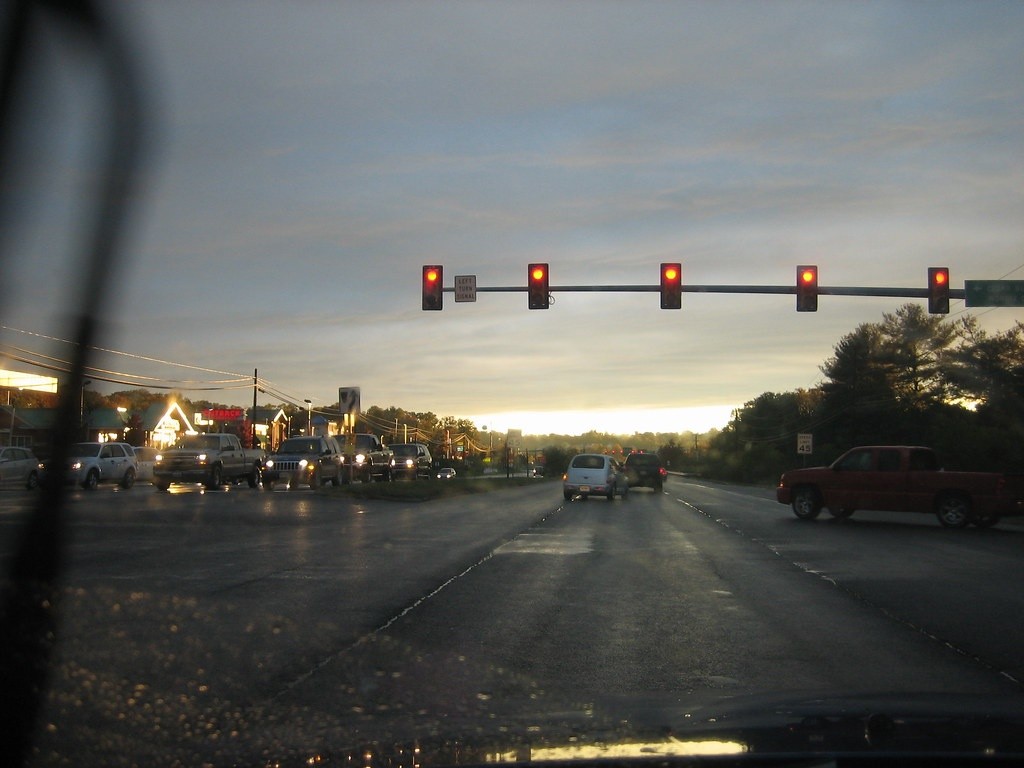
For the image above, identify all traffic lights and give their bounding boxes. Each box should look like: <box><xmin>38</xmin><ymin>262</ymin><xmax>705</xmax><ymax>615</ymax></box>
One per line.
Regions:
<box><xmin>527</xmin><ymin>262</ymin><xmax>550</xmax><ymax>310</ymax></box>
<box><xmin>926</xmin><ymin>265</ymin><xmax>951</xmax><ymax>316</ymax></box>
<box><xmin>660</xmin><ymin>261</ymin><xmax>683</xmax><ymax>310</ymax></box>
<box><xmin>422</xmin><ymin>264</ymin><xmax>443</xmax><ymax>311</ymax></box>
<box><xmin>797</xmin><ymin>264</ymin><xmax>818</xmax><ymax>313</ymax></box>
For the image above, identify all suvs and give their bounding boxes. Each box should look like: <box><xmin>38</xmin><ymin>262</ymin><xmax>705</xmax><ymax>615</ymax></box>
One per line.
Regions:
<box><xmin>264</xmin><ymin>434</ymin><xmax>346</xmax><ymax>488</ymax></box>
<box><xmin>387</xmin><ymin>443</ymin><xmax>432</xmax><ymax>481</ymax></box>
<box><xmin>619</xmin><ymin>452</ymin><xmax>668</xmax><ymax>494</ymax></box>
<box><xmin>329</xmin><ymin>432</ymin><xmax>395</xmax><ymax>484</ymax></box>
<box><xmin>60</xmin><ymin>441</ymin><xmax>138</xmax><ymax>491</ymax></box>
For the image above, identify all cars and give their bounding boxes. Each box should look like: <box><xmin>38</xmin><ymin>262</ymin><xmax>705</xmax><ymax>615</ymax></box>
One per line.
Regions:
<box><xmin>436</xmin><ymin>468</ymin><xmax>457</xmax><ymax>480</ymax></box>
<box><xmin>533</xmin><ymin>467</ymin><xmax>545</xmax><ymax>477</ymax></box>
<box><xmin>131</xmin><ymin>446</ymin><xmax>162</xmax><ymax>483</ymax></box>
<box><xmin>0</xmin><ymin>445</ymin><xmax>45</xmax><ymax>490</ymax></box>
<box><xmin>561</xmin><ymin>454</ymin><xmax>621</xmax><ymax>502</ymax></box>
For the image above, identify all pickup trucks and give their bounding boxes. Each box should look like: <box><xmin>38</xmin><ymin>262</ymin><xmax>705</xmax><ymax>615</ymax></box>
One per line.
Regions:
<box><xmin>777</xmin><ymin>444</ymin><xmax>1024</xmax><ymax>528</ymax></box>
<box><xmin>153</xmin><ymin>432</ymin><xmax>265</xmax><ymax>491</ymax></box>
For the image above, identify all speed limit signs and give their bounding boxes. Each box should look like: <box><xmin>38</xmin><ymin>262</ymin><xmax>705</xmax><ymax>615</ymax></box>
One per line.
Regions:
<box><xmin>797</xmin><ymin>433</ymin><xmax>814</xmax><ymax>453</ymax></box>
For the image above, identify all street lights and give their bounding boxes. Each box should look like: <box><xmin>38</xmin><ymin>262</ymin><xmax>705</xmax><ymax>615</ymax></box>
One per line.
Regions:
<box><xmin>403</xmin><ymin>423</ymin><xmax>407</xmax><ymax>444</ymax></box>
<box><xmin>394</xmin><ymin>417</ymin><xmax>398</xmax><ymax>435</ymax></box>
<box><xmin>304</xmin><ymin>399</ymin><xmax>311</xmax><ymax>433</ymax></box>
<box><xmin>78</xmin><ymin>380</ymin><xmax>91</xmax><ymax>429</ymax></box>
